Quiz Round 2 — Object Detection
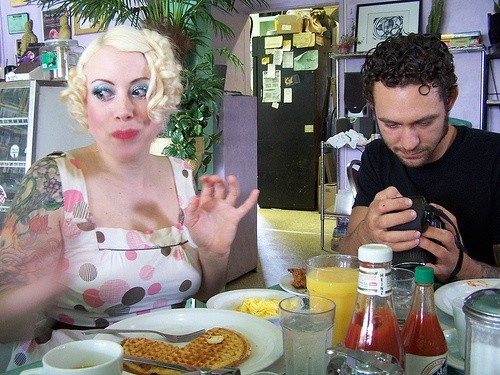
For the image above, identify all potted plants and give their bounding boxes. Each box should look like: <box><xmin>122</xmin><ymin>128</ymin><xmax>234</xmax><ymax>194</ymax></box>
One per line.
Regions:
<box><xmin>488</xmin><ymin>0</ymin><xmax>500</xmax><ymax>45</ymax></box>
<box><xmin>340</xmin><ymin>25</ymin><xmax>363</xmax><ymax>54</ymax></box>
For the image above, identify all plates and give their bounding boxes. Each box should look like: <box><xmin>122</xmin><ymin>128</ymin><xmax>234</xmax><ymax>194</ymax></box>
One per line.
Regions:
<box><xmin>432</xmin><ymin>279</ymin><xmax>500</xmax><ymax>320</ymax></box>
<box><xmin>438</xmin><ymin>325</ymin><xmax>466</xmax><ymax>371</ymax></box>
<box><xmin>207</xmin><ymin>289</ymin><xmax>305</xmax><ymax>319</ymax></box>
<box><xmin>279</xmin><ymin>271</ymin><xmax>308</xmax><ymax>297</ymax></box>
<box><xmin>93</xmin><ymin>308</ymin><xmax>284</xmax><ymax>375</ymax></box>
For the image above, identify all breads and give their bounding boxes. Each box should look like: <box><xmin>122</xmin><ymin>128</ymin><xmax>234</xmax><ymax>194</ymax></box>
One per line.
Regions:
<box><xmin>119</xmin><ymin>328</ymin><xmax>251</xmax><ymax>375</ymax></box>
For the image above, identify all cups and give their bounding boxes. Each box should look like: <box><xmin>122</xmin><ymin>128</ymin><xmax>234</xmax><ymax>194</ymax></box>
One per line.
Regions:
<box><xmin>278</xmin><ymin>293</ymin><xmax>337</xmax><ymax>375</ymax></box>
<box><xmin>41</xmin><ymin>338</ymin><xmax>124</xmax><ymax>375</ymax></box>
<box><xmin>451</xmin><ymin>295</ymin><xmax>471</xmax><ymax>360</ymax></box>
<box><xmin>303</xmin><ymin>253</ymin><xmax>364</xmax><ymax>349</ymax></box>
<box><xmin>387</xmin><ymin>266</ymin><xmax>416</xmax><ymax>330</ymax></box>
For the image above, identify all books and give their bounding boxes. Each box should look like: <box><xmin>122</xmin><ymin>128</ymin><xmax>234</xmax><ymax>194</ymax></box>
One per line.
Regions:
<box><xmin>441</xmin><ymin>30</ymin><xmax>481</xmax><ymax>48</ymax></box>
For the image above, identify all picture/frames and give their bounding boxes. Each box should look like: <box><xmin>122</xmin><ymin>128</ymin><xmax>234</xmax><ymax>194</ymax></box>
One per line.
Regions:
<box><xmin>42</xmin><ymin>9</ymin><xmax>72</xmax><ymax>42</ymax></box>
<box><xmin>353</xmin><ymin>0</ymin><xmax>423</xmax><ymax>53</ymax></box>
<box><xmin>74</xmin><ymin>6</ymin><xmax>108</xmax><ymax>34</ymax></box>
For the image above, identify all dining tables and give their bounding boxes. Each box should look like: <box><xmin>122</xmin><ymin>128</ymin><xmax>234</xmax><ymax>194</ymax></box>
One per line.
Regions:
<box><xmin>5</xmin><ymin>262</ymin><xmax>464</xmax><ymax>375</ymax></box>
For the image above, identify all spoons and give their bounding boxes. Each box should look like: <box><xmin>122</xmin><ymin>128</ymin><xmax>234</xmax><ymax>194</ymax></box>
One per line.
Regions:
<box><xmin>121</xmin><ymin>353</ymin><xmax>243</xmax><ymax>374</ymax></box>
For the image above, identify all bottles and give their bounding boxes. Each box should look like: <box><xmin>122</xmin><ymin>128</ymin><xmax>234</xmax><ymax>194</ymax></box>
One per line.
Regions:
<box><xmin>461</xmin><ymin>289</ymin><xmax>500</xmax><ymax>375</ymax></box>
<box><xmin>341</xmin><ymin>242</ymin><xmax>407</xmax><ymax>372</ymax></box>
<box><xmin>400</xmin><ymin>265</ymin><xmax>449</xmax><ymax>375</ymax></box>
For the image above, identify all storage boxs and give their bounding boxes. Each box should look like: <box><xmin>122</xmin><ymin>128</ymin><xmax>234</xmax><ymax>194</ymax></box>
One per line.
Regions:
<box><xmin>277</xmin><ymin>15</ymin><xmax>303</xmax><ymax>34</ymax></box>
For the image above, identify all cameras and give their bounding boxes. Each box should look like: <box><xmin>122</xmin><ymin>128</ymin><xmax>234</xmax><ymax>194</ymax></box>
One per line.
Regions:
<box><xmin>385</xmin><ymin>196</ymin><xmax>443</xmax><ymax>273</ymax></box>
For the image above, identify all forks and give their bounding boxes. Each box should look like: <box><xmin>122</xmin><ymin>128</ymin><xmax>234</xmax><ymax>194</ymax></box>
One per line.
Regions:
<box><xmin>81</xmin><ymin>326</ymin><xmax>206</xmax><ymax>344</ymax></box>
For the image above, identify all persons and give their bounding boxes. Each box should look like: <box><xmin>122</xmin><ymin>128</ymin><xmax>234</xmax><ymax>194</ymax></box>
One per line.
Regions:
<box><xmin>0</xmin><ymin>26</ymin><xmax>257</xmax><ymax>372</ymax></box>
<box><xmin>18</xmin><ymin>15</ymin><xmax>71</xmax><ymax>57</ymax></box>
<box><xmin>334</xmin><ymin>34</ymin><xmax>500</xmax><ymax>282</ymax></box>
